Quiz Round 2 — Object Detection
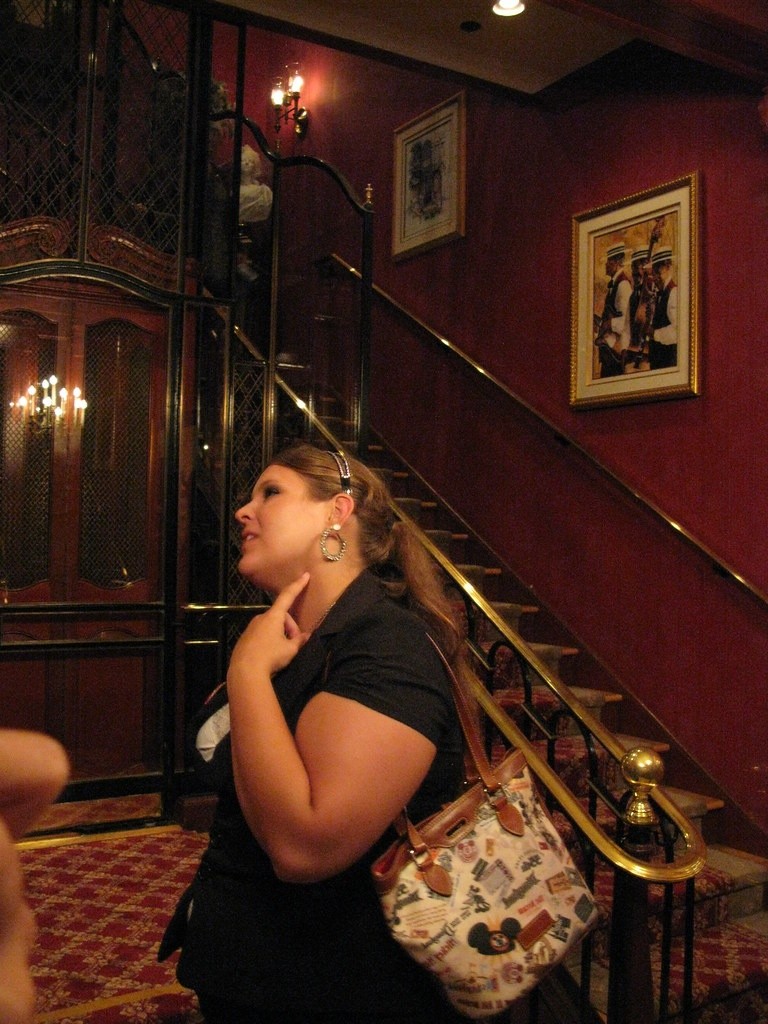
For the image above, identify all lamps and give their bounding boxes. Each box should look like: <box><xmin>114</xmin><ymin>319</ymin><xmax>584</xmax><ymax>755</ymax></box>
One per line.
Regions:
<box><xmin>269</xmin><ymin>70</ymin><xmax>308</xmax><ymax>140</ymax></box>
<box><xmin>17</xmin><ymin>375</ymin><xmax>88</xmax><ymax>438</ymax></box>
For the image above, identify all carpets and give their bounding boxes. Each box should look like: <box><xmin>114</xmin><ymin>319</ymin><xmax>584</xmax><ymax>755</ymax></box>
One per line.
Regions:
<box><xmin>292</xmin><ymin>392</ymin><xmax>767</xmax><ymax>1024</ymax></box>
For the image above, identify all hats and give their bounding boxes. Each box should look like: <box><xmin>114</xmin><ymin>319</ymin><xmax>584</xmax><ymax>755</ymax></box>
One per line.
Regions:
<box><xmin>622</xmin><ymin>245</ymin><xmax>653</xmax><ymax>265</ymax></box>
<box><xmin>599</xmin><ymin>242</ymin><xmax>633</xmax><ymax>261</ymax></box>
<box><xmin>643</xmin><ymin>246</ymin><xmax>678</xmax><ymax>268</ymax></box>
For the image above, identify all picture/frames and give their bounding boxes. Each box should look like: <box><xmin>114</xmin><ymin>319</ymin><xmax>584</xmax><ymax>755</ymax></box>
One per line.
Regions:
<box><xmin>569</xmin><ymin>169</ymin><xmax>703</xmax><ymax>409</ymax></box>
<box><xmin>389</xmin><ymin>89</ymin><xmax>465</xmax><ymax>264</ymax></box>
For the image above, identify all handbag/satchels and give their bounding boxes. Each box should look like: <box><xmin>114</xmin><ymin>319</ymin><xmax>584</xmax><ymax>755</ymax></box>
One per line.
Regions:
<box><xmin>321</xmin><ymin>618</ymin><xmax>599</xmax><ymax>1019</ymax></box>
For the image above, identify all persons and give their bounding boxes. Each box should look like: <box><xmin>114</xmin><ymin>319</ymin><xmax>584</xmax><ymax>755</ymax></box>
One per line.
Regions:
<box><xmin>594</xmin><ymin>242</ymin><xmax>678</xmax><ymax>378</ymax></box>
<box><xmin>1</xmin><ymin>730</ymin><xmax>71</xmax><ymax>1024</ymax></box>
<box><xmin>156</xmin><ymin>443</ymin><xmax>604</xmax><ymax>1024</ymax></box>
<box><xmin>239</xmin><ymin>143</ymin><xmax>273</xmax><ymax>224</ymax></box>
